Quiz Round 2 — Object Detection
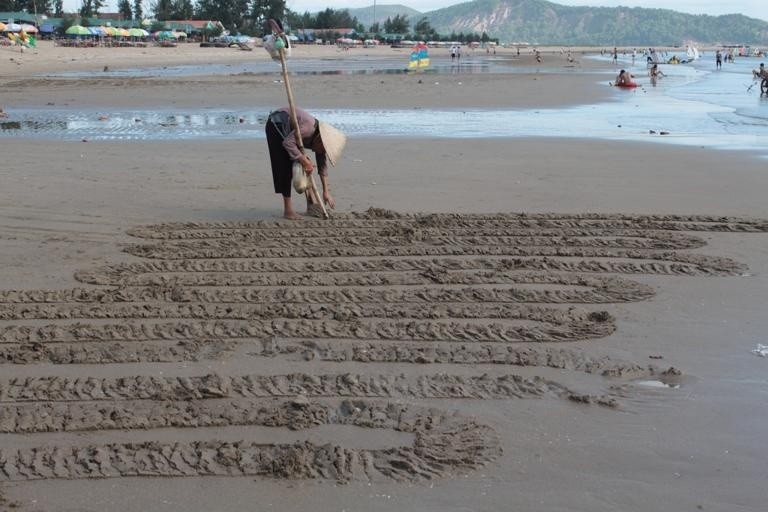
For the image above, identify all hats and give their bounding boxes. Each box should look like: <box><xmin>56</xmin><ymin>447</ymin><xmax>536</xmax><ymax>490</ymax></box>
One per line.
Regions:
<box><xmin>318</xmin><ymin>119</ymin><xmax>347</xmax><ymax>170</ymax></box>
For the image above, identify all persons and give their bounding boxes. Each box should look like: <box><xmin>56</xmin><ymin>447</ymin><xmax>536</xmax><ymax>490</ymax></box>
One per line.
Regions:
<box><xmin>449</xmin><ymin>46</ymin><xmax>578</xmax><ymax>64</ymax></box>
<box><xmin>598</xmin><ymin>40</ymin><xmax>768</xmax><ymax>89</ymax></box>
<box><xmin>264</xmin><ymin>104</ymin><xmax>346</xmax><ymax>222</ymax></box>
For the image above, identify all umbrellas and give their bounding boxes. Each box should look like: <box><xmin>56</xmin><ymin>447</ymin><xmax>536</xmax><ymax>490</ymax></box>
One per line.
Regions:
<box><xmin>0</xmin><ymin>23</ymin><xmax>190</xmax><ymax>48</ymax></box>
